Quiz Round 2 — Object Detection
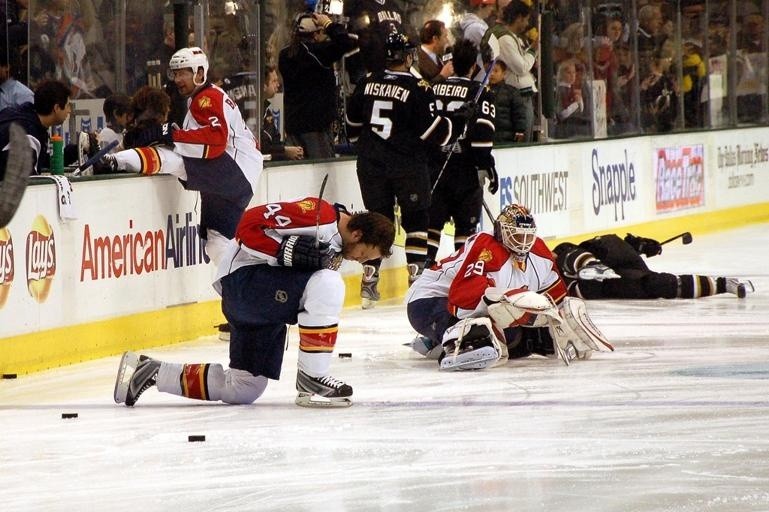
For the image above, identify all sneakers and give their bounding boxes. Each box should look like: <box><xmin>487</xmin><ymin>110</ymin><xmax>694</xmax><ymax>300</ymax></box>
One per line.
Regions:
<box><xmin>360</xmin><ymin>265</ymin><xmax>380</xmax><ymax>301</ymax></box>
<box><xmin>89</xmin><ymin>131</ymin><xmax>118</xmax><ymax>175</ymax></box>
<box><xmin>577</xmin><ymin>261</ymin><xmax>615</xmax><ymax>280</ymax></box>
<box><xmin>407</xmin><ymin>264</ymin><xmax>420</xmax><ymax>287</ymax></box>
<box><xmin>219</xmin><ymin>323</ymin><xmax>233</xmax><ymax>332</ymax></box>
<box><xmin>126</xmin><ymin>355</ymin><xmax>162</xmax><ymax>407</ymax></box>
<box><xmin>725</xmin><ymin>277</ymin><xmax>746</xmax><ymax>298</ymax></box>
<box><xmin>438</xmin><ymin>337</ymin><xmax>494</xmax><ymax>367</ymax></box>
<box><xmin>296</xmin><ymin>369</ymin><xmax>353</xmax><ymax>398</ymax></box>
<box><xmin>424</xmin><ymin>259</ymin><xmax>437</xmax><ymax>268</ymax></box>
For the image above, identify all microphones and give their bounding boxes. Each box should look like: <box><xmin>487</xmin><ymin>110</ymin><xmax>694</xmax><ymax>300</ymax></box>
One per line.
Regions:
<box><xmin>442</xmin><ymin>47</ymin><xmax>452</xmax><ymax>65</ymax></box>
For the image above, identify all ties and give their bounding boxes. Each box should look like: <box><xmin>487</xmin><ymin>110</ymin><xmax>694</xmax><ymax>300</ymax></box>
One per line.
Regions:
<box><xmin>437</xmin><ymin>57</ymin><xmax>442</xmax><ymax>69</ymax></box>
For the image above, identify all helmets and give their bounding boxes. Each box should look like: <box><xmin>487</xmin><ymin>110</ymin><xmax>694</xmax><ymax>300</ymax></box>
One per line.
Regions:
<box><xmin>170</xmin><ymin>47</ymin><xmax>209</xmax><ymax>86</ymax></box>
<box><xmin>494</xmin><ymin>204</ymin><xmax>537</xmax><ymax>255</ymax></box>
<box><xmin>385</xmin><ymin>34</ymin><xmax>418</xmax><ymax>68</ymax></box>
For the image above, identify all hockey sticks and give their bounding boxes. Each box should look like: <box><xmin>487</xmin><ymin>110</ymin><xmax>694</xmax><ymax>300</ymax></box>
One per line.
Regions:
<box><xmin>638</xmin><ymin>232</ymin><xmax>693</xmax><ymax>255</ymax></box>
<box><xmin>315</xmin><ymin>172</ymin><xmax>329</xmax><ymax>247</ymax></box>
<box><xmin>431</xmin><ymin>60</ymin><xmax>495</xmax><ymax>192</ymax></box>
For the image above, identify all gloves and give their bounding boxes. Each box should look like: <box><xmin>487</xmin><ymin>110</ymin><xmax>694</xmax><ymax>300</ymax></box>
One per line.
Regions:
<box><xmin>624</xmin><ymin>233</ymin><xmax>662</xmax><ymax>258</ymax></box>
<box><xmin>278</xmin><ymin>234</ymin><xmax>335</xmax><ymax>270</ymax></box>
<box><xmin>475</xmin><ymin>156</ymin><xmax>499</xmax><ymax>195</ymax></box>
<box><xmin>446</xmin><ymin>101</ymin><xmax>478</xmax><ymax>147</ymax></box>
<box><xmin>350</xmin><ymin>138</ymin><xmax>361</xmax><ymax>148</ymax></box>
<box><xmin>157</xmin><ymin>122</ymin><xmax>176</xmax><ymax>151</ymax></box>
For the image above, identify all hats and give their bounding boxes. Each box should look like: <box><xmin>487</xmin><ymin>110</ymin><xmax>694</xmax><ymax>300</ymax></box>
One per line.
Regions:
<box><xmin>298</xmin><ymin>18</ymin><xmax>325</xmax><ymax>32</ymax></box>
<box><xmin>470</xmin><ymin>0</ymin><xmax>496</xmax><ymax>7</ymax></box>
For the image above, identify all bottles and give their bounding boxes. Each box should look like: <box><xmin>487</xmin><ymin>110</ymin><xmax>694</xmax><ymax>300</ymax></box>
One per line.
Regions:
<box><xmin>50</xmin><ymin>134</ymin><xmax>64</xmax><ymax>175</ymax></box>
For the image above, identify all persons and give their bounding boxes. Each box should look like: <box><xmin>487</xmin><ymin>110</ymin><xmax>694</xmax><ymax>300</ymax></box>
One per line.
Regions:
<box><xmin>316</xmin><ymin>0</ymin><xmax>412</xmax><ymax>34</ymax></box>
<box><xmin>113</xmin><ymin>198</ymin><xmax>395</xmax><ymax>408</ymax></box>
<box><xmin>452</xmin><ymin>0</ymin><xmax>496</xmax><ymax>82</ymax></box>
<box><xmin>490</xmin><ymin>0</ymin><xmax>541</xmax><ymax>139</ymax></box>
<box><xmin>0</xmin><ymin>0</ymin><xmax>114</xmax><ymax>94</ymax></box>
<box><xmin>252</xmin><ymin>66</ymin><xmax>303</xmax><ymax>160</ymax></box>
<box><xmin>124</xmin><ymin>87</ymin><xmax>171</xmax><ymax>147</ymax></box>
<box><xmin>402</xmin><ymin>203</ymin><xmax>522</xmax><ymax>371</ymax></box>
<box><xmin>0</xmin><ymin>46</ymin><xmax>35</xmax><ymax>109</ymax></box>
<box><xmin>540</xmin><ymin>0</ymin><xmax>769</xmax><ymax>136</ymax></box>
<box><xmin>123</xmin><ymin>0</ymin><xmax>175</xmax><ymax>87</ymax></box>
<box><xmin>276</xmin><ymin>10</ymin><xmax>352</xmax><ymax>158</ymax></box>
<box><xmin>0</xmin><ymin>80</ymin><xmax>73</xmax><ymax>183</ymax></box>
<box><xmin>170</xmin><ymin>0</ymin><xmax>313</xmax><ymax>55</ymax></box>
<box><xmin>78</xmin><ymin>47</ymin><xmax>263</xmax><ymax>340</ymax></box>
<box><xmin>345</xmin><ymin>34</ymin><xmax>427</xmax><ymax>309</ymax></box>
<box><xmin>407</xmin><ymin>20</ymin><xmax>453</xmax><ymax>84</ymax></box>
<box><xmin>448</xmin><ymin>207</ymin><xmax>614</xmax><ymax>364</ymax></box>
<box><xmin>98</xmin><ymin>94</ymin><xmax>133</xmax><ymax>156</ymax></box>
<box><xmin>485</xmin><ymin>60</ymin><xmax>526</xmax><ymax>145</ymax></box>
<box><xmin>550</xmin><ymin>235</ymin><xmax>755</xmax><ymax>298</ymax></box>
<box><xmin>425</xmin><ymin>39</ymin><xmax>498</xmax><ymax>266</ymax></box>
